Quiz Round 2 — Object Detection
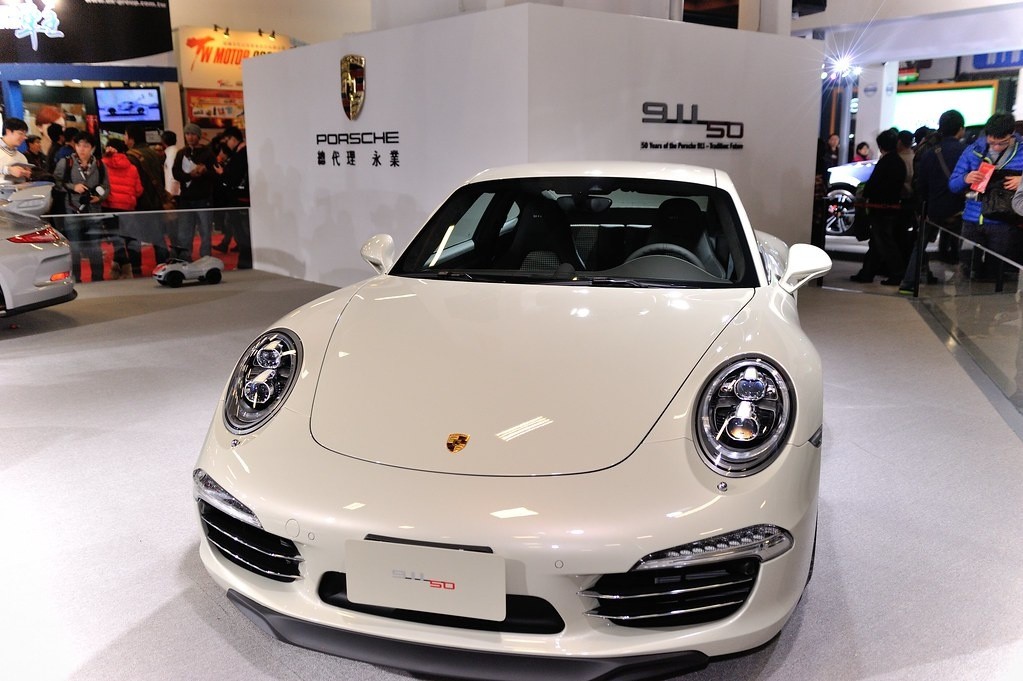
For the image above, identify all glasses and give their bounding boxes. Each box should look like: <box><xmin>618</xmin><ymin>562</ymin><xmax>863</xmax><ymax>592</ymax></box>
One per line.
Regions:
<box><xmin>987</xmin><ymin>138</ymin><xmax>1010</xmax><ymax>146</ymax></box>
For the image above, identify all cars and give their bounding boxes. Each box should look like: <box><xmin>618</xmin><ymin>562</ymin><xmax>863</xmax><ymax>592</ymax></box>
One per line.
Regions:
<box><xmin>105</xmin><ymin>101</ymin><xmax>149</xmax><ymax>116</ymax></box>
<box><xmin>0</xmin><ymin>205</ymin><xmax>79</xmax><ymax>320</ymax></box>
<box><xmin>825</xmin><ymin>160</ymin><xmax>879</xmax><ymax>234</ymax></box>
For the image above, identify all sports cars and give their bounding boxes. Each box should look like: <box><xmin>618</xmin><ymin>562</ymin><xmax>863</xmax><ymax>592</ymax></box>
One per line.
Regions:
<box><xmin>193</xmin><ymin>161</ymin><xmax>833</xmax><ymax>680</ymax></box>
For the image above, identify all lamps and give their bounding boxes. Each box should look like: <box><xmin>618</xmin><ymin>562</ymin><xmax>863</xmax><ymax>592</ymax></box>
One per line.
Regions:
<box><xmin>270</xmin><ymin>30</ymin><xmax>276</xmax><ymax>39</ymax></box>
<box><xmin>258</xmin><ymin>28</ymin><xmax>263</xmax><ymax>37</ymax></box>
<box><xmin>214</xmin><ymin>24</ymin><xmax>221</xmax><ymax>31</ymax></box>
<box><xmin>223</xmin><ymin>26</ymin><xmax>230</xmax><ymax>37</ymax></box>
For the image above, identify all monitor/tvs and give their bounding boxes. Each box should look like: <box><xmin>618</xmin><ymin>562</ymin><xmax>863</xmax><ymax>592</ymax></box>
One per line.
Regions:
<box><xmin>93</xmin><ymin>87</ymin><xmax>164</xmax><ymax>129</ymax></box>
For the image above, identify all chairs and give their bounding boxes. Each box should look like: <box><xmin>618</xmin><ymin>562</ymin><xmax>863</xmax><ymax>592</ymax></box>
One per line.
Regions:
<box><xmin>493</xmin><ymin>198</ymin><xmax>588</xmax><ymax>271</ymax></box>
<box><xmin>638</xmin><ymin>198</ymin><xmax>725</xmax><ymax>279</ymax></box>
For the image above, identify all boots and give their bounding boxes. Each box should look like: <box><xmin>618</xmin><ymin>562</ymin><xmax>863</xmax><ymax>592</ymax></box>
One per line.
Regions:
<box><xmin>118</xmin><ymin>263</ymin><xmax>134</xmax><ymax>279</ymax></box>
<box><xmin>109</xmin><ymin>261</ymin><xmax>120</xmax><ymax>279</ymax></box>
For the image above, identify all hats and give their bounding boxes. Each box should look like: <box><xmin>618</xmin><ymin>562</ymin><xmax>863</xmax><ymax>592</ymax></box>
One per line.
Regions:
<box><xmin>937</xmin><ymin>110</ymin><xmax>963</xmax><ymax>136</ymax></box>
<box><xmin>184</xmin><ymin>124</ymin><xmax>202</xmax><ymax>139</ymax></box>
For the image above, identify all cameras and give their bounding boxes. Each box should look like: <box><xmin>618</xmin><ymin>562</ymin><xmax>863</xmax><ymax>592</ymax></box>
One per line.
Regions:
<box><xmin>79</xmin><ymin>185</ymin><xmax>106</xmax><ymax>205</ymax></box>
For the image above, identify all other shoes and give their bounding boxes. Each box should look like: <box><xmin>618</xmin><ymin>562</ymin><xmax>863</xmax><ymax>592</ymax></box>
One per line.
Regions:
<box><xmin>922</xmin><ymin>274</ymin><xmax>937</xmax><ymax>285</ymax></box>
<box><xmin>880</xmin><ymin>275</ymin><xmax>901</xmax><ymax>285</ymax></box>
<box><xmin>212</xmin><ymin>244</ymin><xmax>228</xmax><ymax>253</ymax></box>
<box><xmin>231</xmin><ymin>245</ymin><xmax>245</xmax><ymax>253</ymax></box>
<box><xmin>132</xmin><ymin>267</ymin><xmax>144</xmax><ymax>278</ymax></box>
<box><xmin>849</xmin><ymin>269</ymin><xmax>873</xmax><ymax>283</ymax></box>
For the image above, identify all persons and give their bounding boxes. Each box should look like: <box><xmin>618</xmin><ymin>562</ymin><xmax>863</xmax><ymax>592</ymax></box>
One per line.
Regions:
<box><xmin>0</xmin><ymin>117</ymin><xmax>252</xmax><ymax>284</ymax></box>
<box><xmin>816</xmin><ymin>110</ymin><xmax>1023</xmax><ymax>295</ymax></box>
<box><xmin>171</xmin><ymin>122</ymin><xmax>217</xmax><ymax>260</ymax></box>
<box><xmin>53</xmin><ymin>131</ymin><xmax>111</xmax><ymax>283</ymax></box>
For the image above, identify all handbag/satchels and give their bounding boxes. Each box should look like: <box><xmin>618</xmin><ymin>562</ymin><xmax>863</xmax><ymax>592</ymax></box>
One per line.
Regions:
<box><xmin>980</xmin><ymin>169</ymin><xmax>1023</xmax><ymax>221</ymax></box>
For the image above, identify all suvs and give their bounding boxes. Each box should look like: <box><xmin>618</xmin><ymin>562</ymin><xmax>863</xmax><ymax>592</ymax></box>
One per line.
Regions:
<box><xmin>153</xmin><ymin>245</ymin><xmax>224</xmax><ymax>289</ymax></box>
